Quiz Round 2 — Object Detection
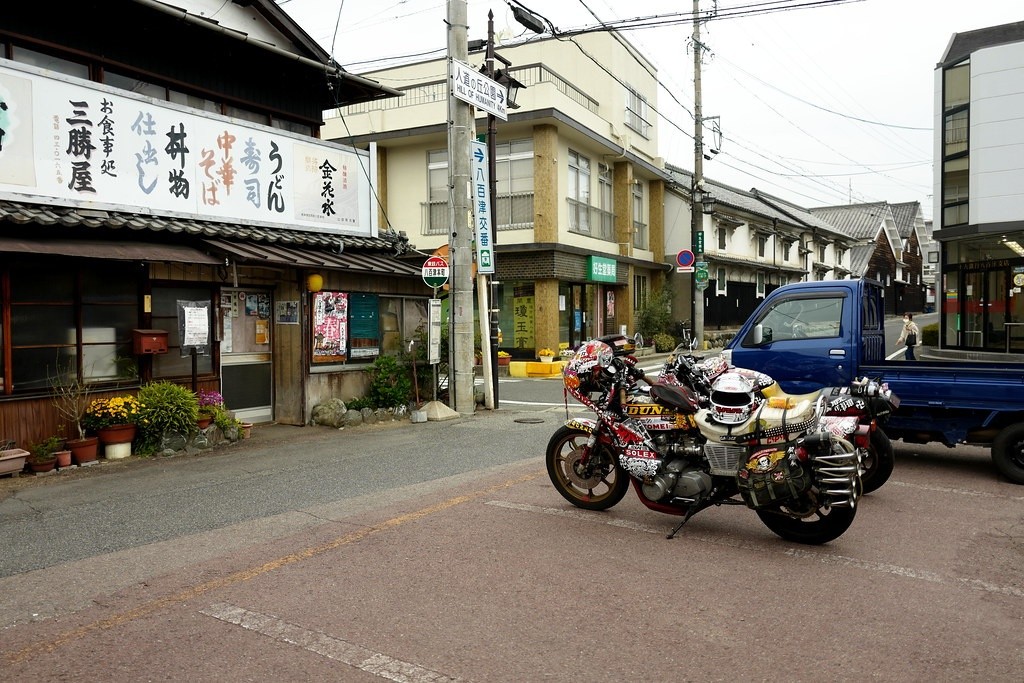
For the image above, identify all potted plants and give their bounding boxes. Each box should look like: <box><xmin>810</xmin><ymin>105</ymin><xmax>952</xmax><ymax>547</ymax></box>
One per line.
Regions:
<box><xmin>474</xmin><ymin>334</ymin><xmax>481</xmax><ymax>365</ymax></box>
<box><xmin>197</xmin><ymin>414</ymin><xmax>212</xmax><ymax>429</ymax></box>
<box><xmin>0</xmin><ymin>439</ymin><xmax>30</xmax><ymax>473</ymax></box>
<box><xmin>47</xmin><ymin>346</ymin><xmax>99</xmax><ymax>463</ymax></box>
<box><xmin>53</xmin><ymin>424</ymin><xmax>72</xmax><ymax>466</ymax></box>
<box><xmin>24</xmin><ymin>434</ymin><xmax>61</xmax><ymax>472</ymax></box>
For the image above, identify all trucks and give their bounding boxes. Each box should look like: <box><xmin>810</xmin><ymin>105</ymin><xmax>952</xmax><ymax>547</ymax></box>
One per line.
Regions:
<box><xmin>722</xmin><ymin>273</ymin><xmax>1023</xmax><ymax>482</ymax></box>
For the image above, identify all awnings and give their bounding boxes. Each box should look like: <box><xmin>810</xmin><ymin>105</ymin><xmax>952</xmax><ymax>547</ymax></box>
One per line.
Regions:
<box><xmin>207</xmin><ymin>238</ymin><xmax>440</xmax><ymax>279</ymax></box>
<box><xmin>0</xmin><ymin>238</ymin><xmax>224</xmax><ymax>266</ymax></box>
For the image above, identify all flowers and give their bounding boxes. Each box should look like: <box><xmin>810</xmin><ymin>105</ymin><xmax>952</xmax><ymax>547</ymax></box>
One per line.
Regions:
<box><xmin>198</xmin><ymin>390</ymin><xmax>225</xmax><ymax>414</ymax></box>
<box><xmin>480</xmin><ymin>350</ymin><xmax>510</xmax><ymax>357</ymax></box>
<box><xmin>82</xmin><ymin>392</ymin><xmax>151</xmax><ymax>428</ymax></box>
<box><xmin>559</xmin><ymin>345</ymin><xmax>580</xmax><ymax>356</ymax></box>
<box><xmin>538</xmin><ymin>347</ymin><xmax>555</xmax><ymax>356</ymax></box>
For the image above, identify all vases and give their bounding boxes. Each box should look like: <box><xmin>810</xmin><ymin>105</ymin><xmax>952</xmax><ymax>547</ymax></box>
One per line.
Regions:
<box><xmin>97</xmin><ymin>423</ymin><xmax>138</xmax><ymax>444</ymax></box>
<box><xmin>480</xmin><ymin>356</ymin><xmax>512</xmax><ymax>365</ymax></box>
<box><xmin>240</xmin><ymin>422</ymin><xmax>253</xmax><ymax>438</ymax></box>
<box><xmin>561</xmin><ymin>356</ymin><xmax>572</xmax><ymax>361</ymax></box>
<box><xmin>540</xmin><ymin>356</ymin><xmax>553</xmax><ymax>362</ymax></box>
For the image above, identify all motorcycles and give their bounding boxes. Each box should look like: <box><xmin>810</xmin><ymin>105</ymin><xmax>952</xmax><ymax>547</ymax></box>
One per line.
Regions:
<box><xmin>657</xmin><ymin>337</ymin><xmax>901</xmax><ymax>497</ymax></box>
<box><xmin>544</xmin><ymin>332</ymin><xmax>861</xmax><ymax>551</ymax></box>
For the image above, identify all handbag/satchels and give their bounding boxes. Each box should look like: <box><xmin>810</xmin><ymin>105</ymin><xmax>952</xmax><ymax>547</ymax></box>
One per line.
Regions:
<box><xmin>905</xmin><ymin>329</ymin><xmax>917</xmax><ymax>347</ymax></box>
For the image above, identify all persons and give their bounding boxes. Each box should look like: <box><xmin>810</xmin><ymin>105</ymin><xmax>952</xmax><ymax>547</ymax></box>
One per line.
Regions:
<box><xmin>896</xmin><ymin>312</ymin><xmax>919</xmax><ymax>360</ymax></box>
